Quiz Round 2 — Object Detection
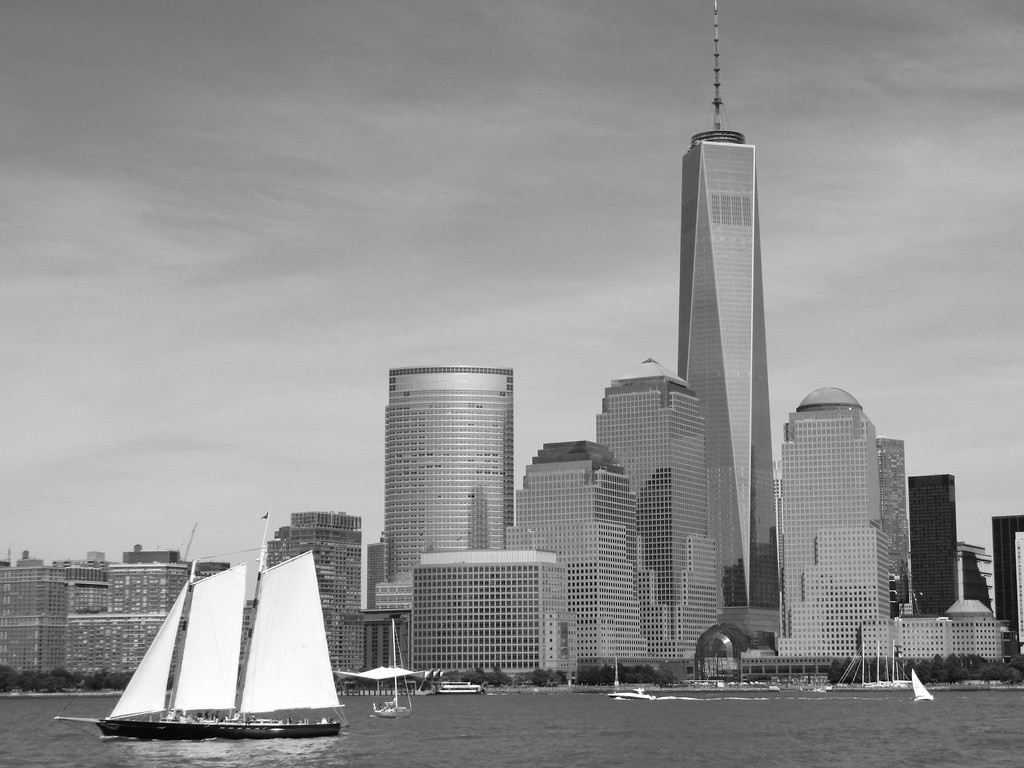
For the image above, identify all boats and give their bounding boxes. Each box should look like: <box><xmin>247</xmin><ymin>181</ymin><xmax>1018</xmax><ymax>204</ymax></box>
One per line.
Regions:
<box><xmin>436</xmin><ymin>682</ymin><xmax>486</xmax><ymax>695</ymax></box>
<box><xmin>370</xmin><ymin>618</ymin><xmax>413</xmax><ymax>718</ymax></box>
<box><xmin>607</xmin><ymin>688</ymin><xmax>655</xmax><ymax>700</ymax></box>
<box><xmin>825</xmin><ymin>640</ymin><xmax>912</xmax><ymax>691</ymax></box>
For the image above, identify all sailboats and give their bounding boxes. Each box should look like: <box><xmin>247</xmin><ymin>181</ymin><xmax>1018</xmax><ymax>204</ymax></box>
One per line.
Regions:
<box><xmin>52</xmin><ymin>509</ymin><xmax>352</xmax><ymax>741</ymax></box>
<box><xmin>911</xmin><ymin>668</ymin><xmax>934</xmax><ymax>701</ymax></box>
